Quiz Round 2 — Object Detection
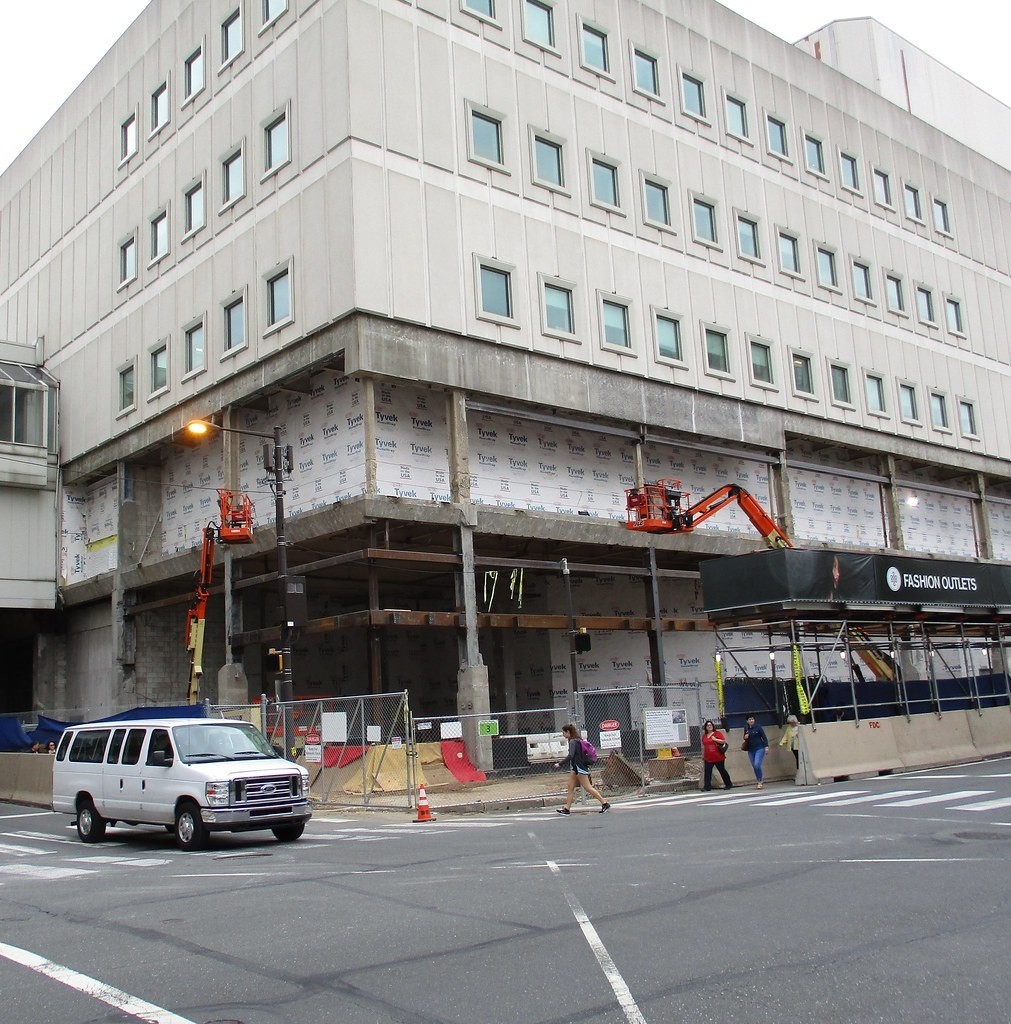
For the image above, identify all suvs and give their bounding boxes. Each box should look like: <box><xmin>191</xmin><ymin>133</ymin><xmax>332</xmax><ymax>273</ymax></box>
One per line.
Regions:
<box><xmin>50</xmin><ymin>716</ymin><xmax>313</xmax><ymax>852</ymax></box>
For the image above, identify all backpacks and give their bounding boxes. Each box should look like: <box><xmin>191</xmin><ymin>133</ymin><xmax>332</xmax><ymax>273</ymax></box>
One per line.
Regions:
<box><xmin>574</xmin><ymin>737</ymin><xmax>597</xmax><ymax>766</ymax></box>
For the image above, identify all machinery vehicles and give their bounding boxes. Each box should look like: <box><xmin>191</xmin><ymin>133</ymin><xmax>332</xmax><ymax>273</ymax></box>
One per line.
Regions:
<box><xmin>626</xmin><ymin>474</ymin><xmax>840</xmax><ymax>602</ymax></box>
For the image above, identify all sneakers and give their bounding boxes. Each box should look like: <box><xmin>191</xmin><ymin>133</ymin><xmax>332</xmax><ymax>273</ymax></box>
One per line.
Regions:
<box><xmin>556</xmin><ymin>808</ymin><xmax>570</xmax><ymax>817</ymax></box>
<box><xmin>598</xmin><ymin>802</ymin><xmax>610</xmax><ymax>813</ymax></box>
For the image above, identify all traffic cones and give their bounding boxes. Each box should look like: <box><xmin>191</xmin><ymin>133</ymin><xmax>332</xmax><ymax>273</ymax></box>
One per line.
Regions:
<box><xmin>411</xmin><ymin>784</ymin><xmax>437</xmax><ymax>823</ymax></box>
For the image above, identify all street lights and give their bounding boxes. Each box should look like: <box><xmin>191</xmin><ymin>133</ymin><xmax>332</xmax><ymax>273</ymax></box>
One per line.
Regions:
<box><xmin>184</xmin><ymin>417</ymin><xmax>299</xmax><ymax>764</ymax></box>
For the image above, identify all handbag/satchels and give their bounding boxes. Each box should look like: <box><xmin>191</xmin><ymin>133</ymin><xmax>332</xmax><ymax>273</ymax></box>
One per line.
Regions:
<box><xmin>714</xmin><ymin>731</ymin><xmax>728</xmax><ymax>754</ymax></box>
<box><xmin>741</xmin><ymin>724</ymin><xmax>749</xmax><ymax>751</ymax></box>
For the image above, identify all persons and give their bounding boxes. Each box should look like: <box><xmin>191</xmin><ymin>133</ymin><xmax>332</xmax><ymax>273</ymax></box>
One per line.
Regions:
<box><xmin>779</xmin><ymin>714</ymin><xmax>806</xmax><ymax>774</ymax></box>
<box><xmin>741</xmin><ymin>714</ymin><xmax>768</xmax><ymax>789</ymax></box>
<box><xmin>27</xmin><ymin>740</ymin><xmax>56</xmax><ymax>754</ymax></box>
<box><xmin>553</xmin><ymin>723</ymin><xmax>611</xmax><ymax>816</ymax></box>
<box><xmin>701</xmin><ymin>721</ymin><xmax>733</xmax><ymax>792</ymax></box>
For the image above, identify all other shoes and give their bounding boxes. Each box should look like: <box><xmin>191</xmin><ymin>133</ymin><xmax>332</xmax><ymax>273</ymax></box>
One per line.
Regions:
<box><xmin>701</xmin><ymin>788</ymin><xmax>711</xmax><ymax>791</ymax></box>
<box><xmin>724</xmin><ymin>785</ymin><xmax>730</xmax><ymax>790</ymax></box>
<box><xmin>757</xmin><ymin>782</ymin><xmax>765</xmax><ymax>789</ymax></box>
